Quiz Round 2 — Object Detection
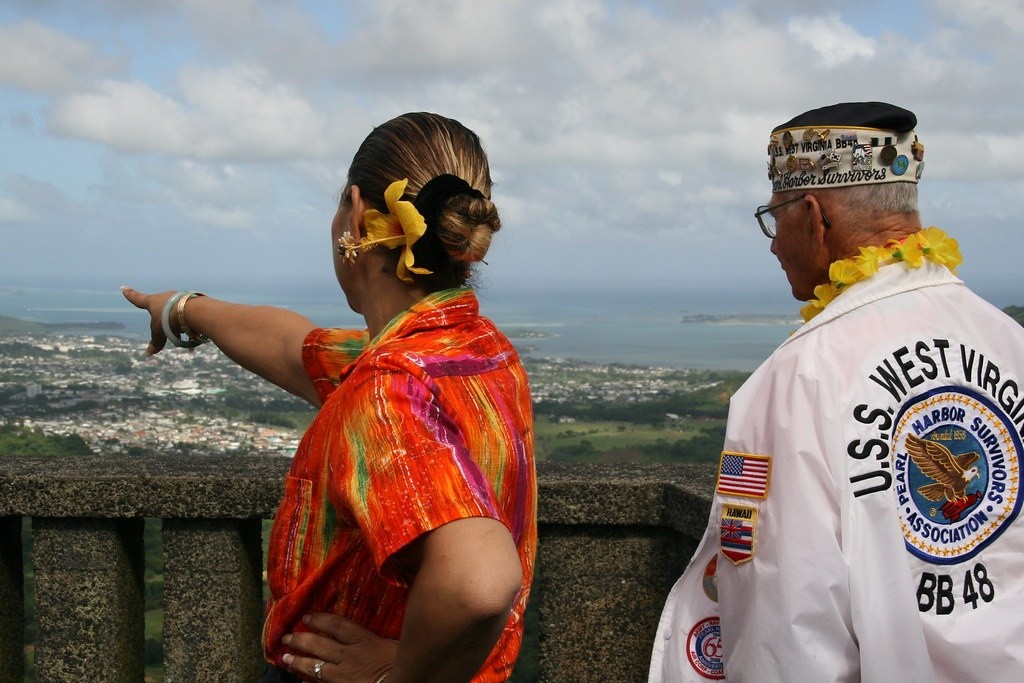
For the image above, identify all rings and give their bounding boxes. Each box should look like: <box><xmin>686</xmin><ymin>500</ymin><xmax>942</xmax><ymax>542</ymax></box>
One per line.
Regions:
<box><xmin>315</xmin><ymin>660</ymin><xmax>326</xmax><ymax>680</ymax></box>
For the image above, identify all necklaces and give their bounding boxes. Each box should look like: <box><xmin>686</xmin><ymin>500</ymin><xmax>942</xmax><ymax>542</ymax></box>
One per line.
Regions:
<box><xmin>789</xmin><ymin>225</ymin><xmax>963</xmax><ymax>337</ymax></box>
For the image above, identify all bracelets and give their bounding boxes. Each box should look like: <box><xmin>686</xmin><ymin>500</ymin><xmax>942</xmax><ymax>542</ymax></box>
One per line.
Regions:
<box><xmin>160</xmin><ymin>290</ymin><xmax>209</xmax><ymax>349</ymax></box>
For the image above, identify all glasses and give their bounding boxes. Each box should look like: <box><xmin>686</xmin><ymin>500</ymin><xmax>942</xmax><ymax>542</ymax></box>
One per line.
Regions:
<box><xmin>754</xmin><ymin>194</ymin><xmax>832</xmax><ymax>238</ymax></box>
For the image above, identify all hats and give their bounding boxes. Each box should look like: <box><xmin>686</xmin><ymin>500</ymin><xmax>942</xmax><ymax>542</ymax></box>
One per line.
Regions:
<box><xmin>767</xmin><ymin>102</ymin><xmax>924</xmax><ymax>193</ymax></box>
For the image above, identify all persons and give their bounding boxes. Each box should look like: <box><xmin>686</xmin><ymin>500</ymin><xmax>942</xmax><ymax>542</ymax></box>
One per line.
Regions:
<box><xmin>646</xmin><ymin>103</ymin><xmax>1024</xmax><ymax>683</ymax></box>
<box><xmin>120</xmin><ymin>111</ymin><xmax>539</xmax><ymax>683</ymax></box>
